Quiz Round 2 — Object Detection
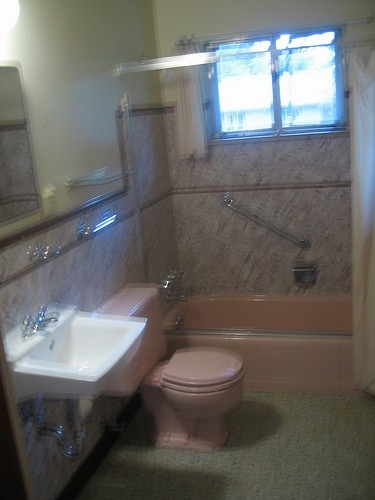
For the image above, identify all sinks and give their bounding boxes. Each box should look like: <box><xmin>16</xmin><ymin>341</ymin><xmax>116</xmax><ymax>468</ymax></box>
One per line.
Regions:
<box><xmin>6</xmin><ymin>312</ymin><xmax>148</xmax><ymax>405</ymax></box>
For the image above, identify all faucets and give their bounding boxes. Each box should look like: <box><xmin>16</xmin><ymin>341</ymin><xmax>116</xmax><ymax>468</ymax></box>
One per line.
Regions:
<box><xmin>34</xmin><ymin>308</ymin><xmax>58</xmax><ymax>331</ymax></box>
<box><xmin>166</xmin><ymin>289</ymin><xmax>187</xmax><ymax>304</ymax></box>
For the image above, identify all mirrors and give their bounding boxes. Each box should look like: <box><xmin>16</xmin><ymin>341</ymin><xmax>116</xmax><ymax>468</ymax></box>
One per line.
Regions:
<box><xmin>0</xmin><ymin>61</ymin><xmax>44</xmax><ymax>228</ymax></box>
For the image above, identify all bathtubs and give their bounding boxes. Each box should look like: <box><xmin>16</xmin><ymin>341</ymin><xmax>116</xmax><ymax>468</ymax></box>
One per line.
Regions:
<box><xmin>162</xmin><ymin>292</ymin><xmax>353</xmax><ymax>393</ymax></box>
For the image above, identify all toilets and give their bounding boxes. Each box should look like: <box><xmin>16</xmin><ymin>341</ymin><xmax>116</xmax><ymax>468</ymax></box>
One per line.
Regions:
<box><xmin>93</xmin><ymin>286</ymin><xmax>246</xmax><ymax>450</ymax></box>
